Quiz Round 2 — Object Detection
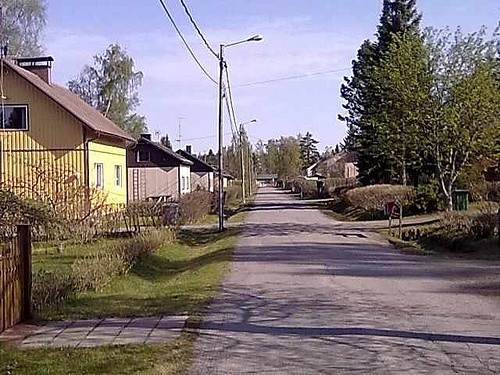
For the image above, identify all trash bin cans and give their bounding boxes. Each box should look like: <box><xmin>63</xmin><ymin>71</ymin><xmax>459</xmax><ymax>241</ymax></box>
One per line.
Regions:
<box><xmin>451</xmin><ymin>190</ymin><xmax>470</xmax><ymax>211</ymax></box>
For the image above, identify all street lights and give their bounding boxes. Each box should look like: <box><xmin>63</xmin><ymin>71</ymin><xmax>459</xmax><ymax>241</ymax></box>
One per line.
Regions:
<box><xmin>218</xmin><ymin>34</ymin><xmax>264</xmax><ymax>234</ymax></box>
<box><xmin>239</xmin><ymin>118</ymin><xmax>258</xmax><ymax>204</ymax></box>
